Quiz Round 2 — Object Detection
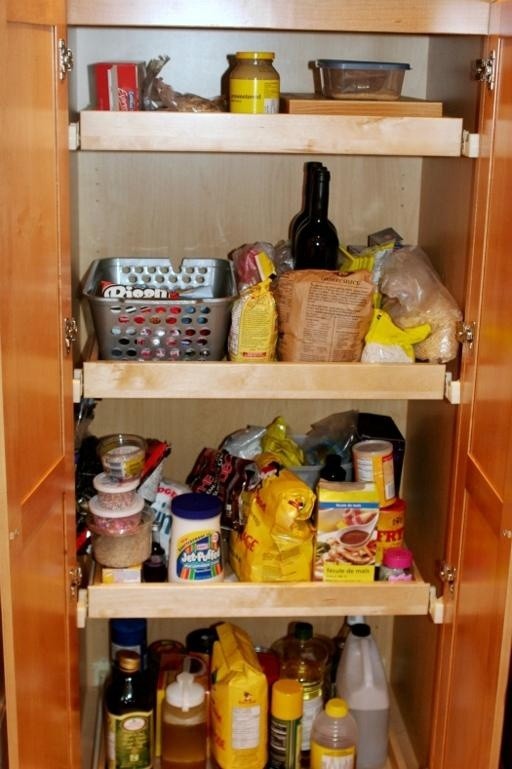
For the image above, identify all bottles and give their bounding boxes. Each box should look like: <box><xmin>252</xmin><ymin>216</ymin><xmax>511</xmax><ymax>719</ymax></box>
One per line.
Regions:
<box><xmin>100</xmin><ymin>650</ymin><xmax>150</xmax><ymax>768</ymax></box>
<box><xmin>318</xmin><ymin>455</ymin><xmax>347</xmax><ymax>481</ymax></box>
<box><xmin>272</xmin><ymin>621</ymin><xmax>356</xmax><ymax>769</ymax></box>
<box><xmin>292</xmin><ymin>166</ymin><xmax>339</xmax><ymax>267</ymax></box>
<box><xmin>138</xmin><ymin>522</ymin><xmax>166</xmax><ymax>583</ymax></box>
<box><xmin>162</xmin><ymin>660</ymin><xmax>207</xmax><ymax>769</ymax></box>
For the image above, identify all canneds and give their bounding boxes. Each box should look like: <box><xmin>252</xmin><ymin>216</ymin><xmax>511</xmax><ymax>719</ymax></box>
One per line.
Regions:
<box><xmin>377</xmin><ymin>500</ymin><xmax>408</xmax><ymax>567</ymax></box>
<box><xmin>354</xmin><ymin>440</ymin><xmax>397</xmax><ymax>507</ymax></box>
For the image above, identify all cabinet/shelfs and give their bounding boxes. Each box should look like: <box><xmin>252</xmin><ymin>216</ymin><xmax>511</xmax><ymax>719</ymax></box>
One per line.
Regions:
<box><xmin>2</xmin><ymin>2</ymin><xmax>512</xmax><ymax>768</ymax></box>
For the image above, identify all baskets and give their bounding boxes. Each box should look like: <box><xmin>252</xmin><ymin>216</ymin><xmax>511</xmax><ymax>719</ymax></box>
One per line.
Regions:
<box><xmin>80</xmin><ymin>253</ymin><xmax>243</xmax><ymax>361</ymax></box>
